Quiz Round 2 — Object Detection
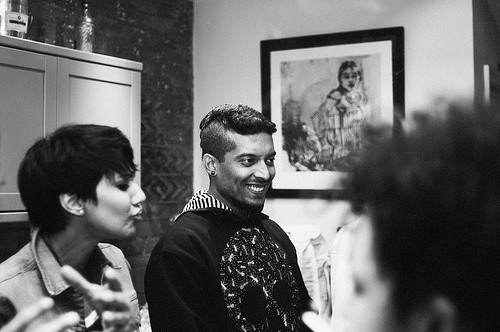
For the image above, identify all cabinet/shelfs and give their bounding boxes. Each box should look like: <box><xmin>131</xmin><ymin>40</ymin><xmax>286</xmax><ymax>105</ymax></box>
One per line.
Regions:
<box><xmin>0</xmin><ymin>35</ymin><xmax>143</xmax><ymax>222</ymax></box>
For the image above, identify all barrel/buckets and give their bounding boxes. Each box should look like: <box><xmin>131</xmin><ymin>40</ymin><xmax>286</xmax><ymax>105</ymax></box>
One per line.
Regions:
<box><xmin>0</xmin><ymin>0</ymin><xmax>30</xmax><ymax>39</ymax></box>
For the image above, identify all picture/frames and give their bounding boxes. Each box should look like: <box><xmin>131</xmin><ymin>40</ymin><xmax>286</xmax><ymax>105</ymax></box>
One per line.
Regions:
<box><xmin>260</xmin><ymin>26</ymin><xmax>405</xmax><ymax>200</ymax></box>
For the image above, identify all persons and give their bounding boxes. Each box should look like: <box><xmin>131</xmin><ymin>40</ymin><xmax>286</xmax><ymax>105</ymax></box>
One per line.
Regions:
<box><xmin>310</xmin><ymin>61</ymin><xmax>371</xmax><ymax>168</ymax></box>
<box><xmin>144</xmin><ymin>104</ymin><xmax>319</xmax><ymax>332</ymax></box>
<box><xmin>0</xmin><ymin>124</ymin><xmax>146</xmax><ymax>332</ymax></box>
<box><xmin>296</xmin><ymin>96</ymin><xmax>500</xmax><ymax>332</ymax></box>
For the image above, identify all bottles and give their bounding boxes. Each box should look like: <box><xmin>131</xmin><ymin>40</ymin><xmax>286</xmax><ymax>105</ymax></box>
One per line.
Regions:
<box><xmin>75</xmin><ymin>0</ymin><xmax>95</xmax><ymax>53</ymax></box>
